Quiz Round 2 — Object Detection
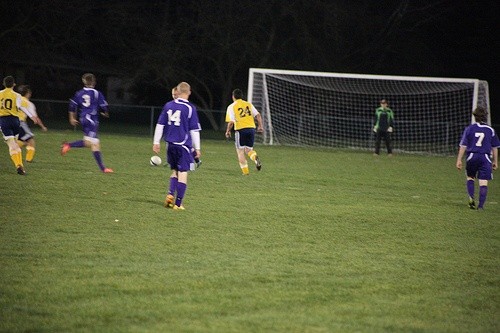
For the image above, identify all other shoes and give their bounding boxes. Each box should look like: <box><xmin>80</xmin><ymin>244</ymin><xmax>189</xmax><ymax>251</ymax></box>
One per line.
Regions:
<box><xmin>17</xmin><ymin>166</ymin><xmax>25</xmax><ymax>175</ymax></box>
<box><xmin>165</xmin><ymin>195</ymin><xmax>173</xmax><ymax>208</ymax></box>
<box><xmin>196</xmin><ymin>160</ymin><xmax>202</xmax><ymax>168</ymax></box>
<box><xmin>59</xmin><ymin>141</ymin><xmax>70</xmax><ymax>155</ymax></box>
<box><xmin>468</xmin><ymin>195</ymin><xmax>476</xmax><ymax>209</ymax></box>
<box><xmin>254</xmin><ymin>155</ymin><xmax>262</xmax><ymax>171</ymax></box>
<box><xmin>174</xmin><ymin>205</ymin><xmax>185</xmax><ymax>210</ymax></box>
<box><xmin>103</xmin><ymin>168</ymin><xmax>113</xmax><ymax>173</ymax></box>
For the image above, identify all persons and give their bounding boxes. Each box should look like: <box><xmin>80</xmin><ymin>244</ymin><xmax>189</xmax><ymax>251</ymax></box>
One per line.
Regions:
<box><xmin>152</xmin><ymin>82</ymin><xmax>202</xmax><ymax>210</ymax></box>
<box><xmin>0</xmin><ymin>76</ymin><xmax>47</xmax><ymax>175</ymax></box>
<box><xmin>226</xmin><ymin>88</ymin><xmax>262</xmax><ymax>176</ymax></box>
<box><xmin>456</xmin><ymin>106</ymin><xmax>500</xmax><ymax>211</ymax></box>
<box><xmin>61</xmin><ymin>74</ymin><xmax>113</xmax><ymax>173</ymax></box>
<box><xmin>373</xmin><ymin>100</ymin><xmax>394</xmax><ymax>158</ymax></box>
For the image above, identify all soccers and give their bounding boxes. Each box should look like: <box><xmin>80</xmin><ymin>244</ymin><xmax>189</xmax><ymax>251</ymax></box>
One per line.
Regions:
<box><xmin>150</xmin><ymin>156</ymin><xmax>161</xmax><ymax>166</ymax></box>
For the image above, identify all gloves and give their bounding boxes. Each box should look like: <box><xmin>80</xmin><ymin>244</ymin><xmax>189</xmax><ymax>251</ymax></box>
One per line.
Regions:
<box><xmin>387</xmin><ymin>127</ymin><xmax>393</xmax><ymax>132</ymax></box>
<box><xmin>373</xmin><ymin>126</ymin><xmax>377</xmax><ymax>133</ymax></box>
